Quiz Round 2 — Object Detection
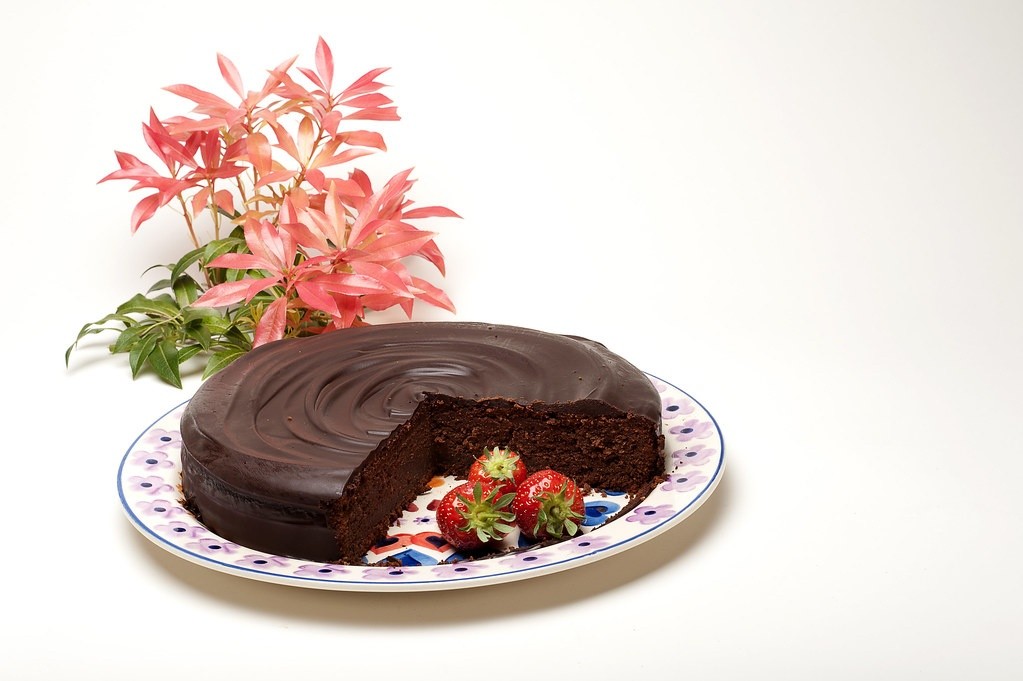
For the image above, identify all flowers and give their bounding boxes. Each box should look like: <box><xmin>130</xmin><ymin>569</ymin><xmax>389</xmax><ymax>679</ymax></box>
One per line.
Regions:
<box><xmin>62</xmin><ymin>37</ymin><xmax>461</xmax><ymax>389</ymax></box>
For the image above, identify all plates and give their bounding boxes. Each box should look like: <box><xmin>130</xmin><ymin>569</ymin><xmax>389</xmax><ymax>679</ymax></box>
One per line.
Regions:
<box><xmin>117</xmin><ymin>334</ymin><xmax>728</xmax><ymax>594</ymax></box>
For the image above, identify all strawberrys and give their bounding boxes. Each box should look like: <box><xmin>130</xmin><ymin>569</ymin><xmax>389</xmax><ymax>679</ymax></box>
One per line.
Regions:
<box><xmin>435</xmin><ymin>445</ymin><xmax>586</xmax><ymax>551</ymax></box>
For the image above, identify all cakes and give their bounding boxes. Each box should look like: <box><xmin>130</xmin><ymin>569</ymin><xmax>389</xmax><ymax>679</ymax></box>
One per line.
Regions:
<box><xmin>179</xmin><ymin>322</ymin><xmax>664</xmax><ymax>565</ymax></box>
